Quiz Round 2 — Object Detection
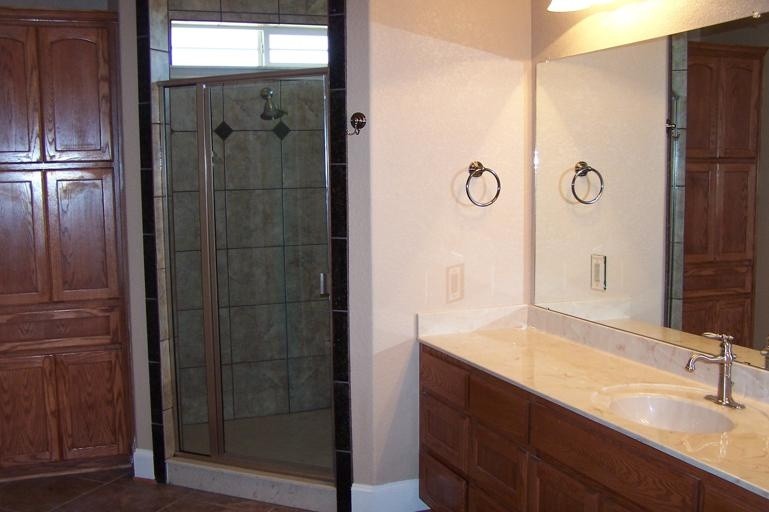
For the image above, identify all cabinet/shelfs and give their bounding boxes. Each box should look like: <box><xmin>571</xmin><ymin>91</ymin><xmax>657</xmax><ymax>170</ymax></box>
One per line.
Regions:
<box><xmin>1</xmin><ymin>7</ymin><xmax>135</xmax><ymax>484</ymax></box>
<box><xmin>416</xmin><ymin>342</ymin><xmax>532</xmax><ymax>512</ymax></box>
<box><xmin>532</xmin><ymin>397</ymin><xmax>768</xmax><ymax>509</ymax></box>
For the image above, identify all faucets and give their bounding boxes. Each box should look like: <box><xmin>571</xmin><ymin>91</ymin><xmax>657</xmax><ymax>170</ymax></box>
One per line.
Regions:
<box><xmin>686</xmin><ymin>333</ymin><xmax>736</xmax><ymax>403</ymax></box>
<box><xmin>761</xmin><ymin>346</ymin><xmax>768</xmax><ymax>368</ymax></box>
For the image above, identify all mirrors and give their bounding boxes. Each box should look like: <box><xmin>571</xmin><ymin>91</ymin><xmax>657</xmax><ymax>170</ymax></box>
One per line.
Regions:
<box><xmin>531</xmin><ymin>7</ymin><xmax>768</xmax><ymax>375</ymax></box>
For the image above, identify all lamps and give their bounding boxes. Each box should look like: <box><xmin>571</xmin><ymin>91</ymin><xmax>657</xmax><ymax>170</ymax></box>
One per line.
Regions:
<box><xmin>259</xmin><ymin>88</ymin><xmax>292</xmax><ymax>121</ymax></box>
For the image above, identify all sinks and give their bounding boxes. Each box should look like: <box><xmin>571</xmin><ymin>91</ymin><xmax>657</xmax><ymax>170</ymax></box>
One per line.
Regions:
<box><xmin>608</xmin><ymin>392</ymin><xmax>735</xmax><ymax>435</ymax></box>
